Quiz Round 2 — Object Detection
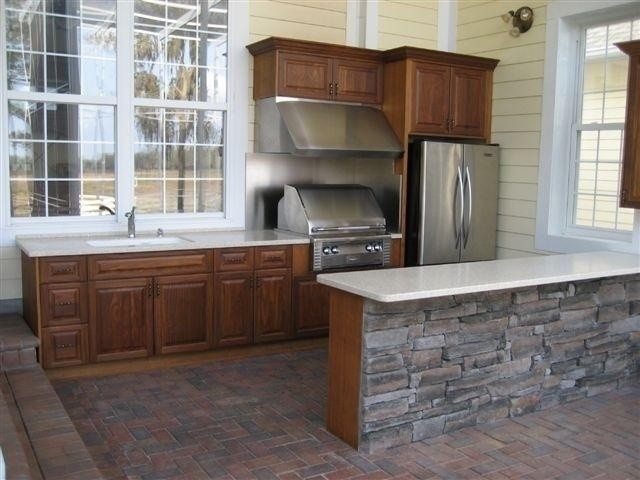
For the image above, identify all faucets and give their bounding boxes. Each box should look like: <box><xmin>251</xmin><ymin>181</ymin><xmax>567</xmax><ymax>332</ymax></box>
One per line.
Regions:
<box><xmin>100</xmin><ymin>206</ymin><xmax>115</xmax><ymax>216</ymax></box>
<box><xmin>125</xmin><ymin>207</ymin><xmax>135</xmax><ymax>237</ymax></box>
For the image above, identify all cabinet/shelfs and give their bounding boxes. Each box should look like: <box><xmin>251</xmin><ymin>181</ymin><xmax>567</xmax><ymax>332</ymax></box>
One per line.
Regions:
<box><xmin>89</xmin><ymin>248</ymin><xmax>213</xmax><ymax>365</ymax></box>
<box><xmin>295</xmin><ymin>273</ymin><xmax>331</xmax><ymax>338</ymax></box>
<box><xmin>383</xmin><ymin>46</ymin><xmax>501</xmax><ymax>138</ymax></box>
<box><xmin>612</xmin><ymin>38</ymin><xmax>639</xmax><ymax>209</ymax></box>
<box><xmin>35</xmin><ymin>253</ymin><xmax>90</xmax><ymax>371</ymax></box>
<box><xmin>212</xmin><ymin>243</ymin><xmax>293</xmax><ymax>344</ymax></box>
<box><xmin>246</xmin><ymin>36</ymin><xmax>384</xmax><ymax>105</ymax></box>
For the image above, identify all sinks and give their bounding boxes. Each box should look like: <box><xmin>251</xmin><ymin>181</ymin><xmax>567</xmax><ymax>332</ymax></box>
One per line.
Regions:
<box><xmin>84</xmin><ymin>237</ymin><xmax>194</xmax><ymax>248</ymax></box>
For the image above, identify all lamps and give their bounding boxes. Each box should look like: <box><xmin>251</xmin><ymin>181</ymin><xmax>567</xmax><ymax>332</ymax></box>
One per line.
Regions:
<box><xmin>501</xmin><ymin>6</ymin><xmax>533</xmax><ymax>38</ymax></box>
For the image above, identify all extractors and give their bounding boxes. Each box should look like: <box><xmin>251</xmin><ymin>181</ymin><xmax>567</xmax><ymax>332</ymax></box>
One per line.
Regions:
<box><xmin>255</xmin><ymin>95</ymin><xmax>405</xmax><ymax>161</ymax></box>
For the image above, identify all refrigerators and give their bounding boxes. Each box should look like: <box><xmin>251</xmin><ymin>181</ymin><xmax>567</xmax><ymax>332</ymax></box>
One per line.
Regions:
<box><xmin>408</xmin><ymin>137</ymin><xmax>500</xmax><ymax>267</ymax></box>
